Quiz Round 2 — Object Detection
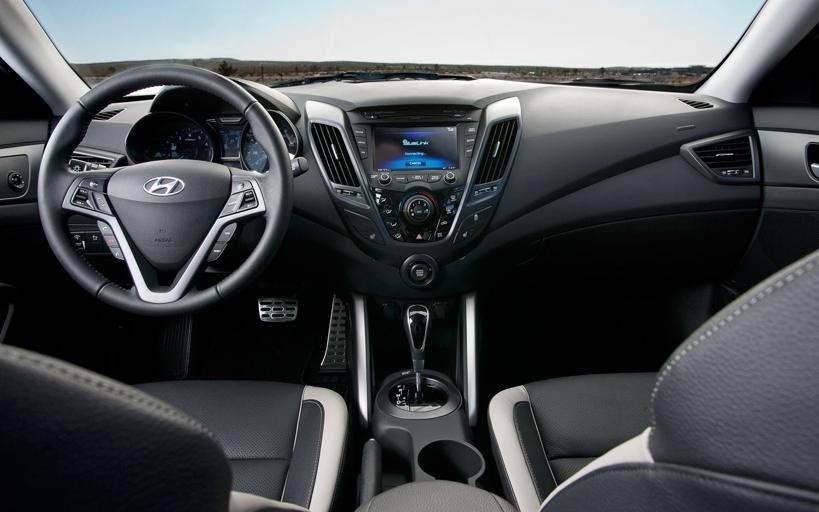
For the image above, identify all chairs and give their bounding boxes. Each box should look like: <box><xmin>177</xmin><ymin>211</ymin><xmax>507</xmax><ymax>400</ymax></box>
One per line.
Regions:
<box><xmin>0</xmin><ymin>343</ymin><xmax>351</xmax><ymax>512</ymax></box>
<box><xmin>488</xmin><ymin>251</ymin><xmax>819</xmax><ymax>511</ymax></box>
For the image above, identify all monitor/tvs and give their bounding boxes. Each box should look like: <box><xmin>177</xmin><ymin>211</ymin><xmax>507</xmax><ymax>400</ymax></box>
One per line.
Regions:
<box><xmin>369</xmin><ymin>123</ymin><xmax>461</xmax><ymax>174</ymax></box>
